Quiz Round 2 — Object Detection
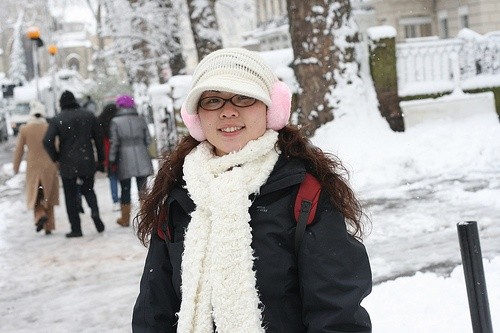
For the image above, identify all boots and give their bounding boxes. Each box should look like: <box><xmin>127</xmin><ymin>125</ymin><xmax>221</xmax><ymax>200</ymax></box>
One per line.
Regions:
<box><xmin>117</xmin><ymin>202</ymin><xmax>132</xmax><ymax>227</ymax></box>
<box><xmin>139</xmin><ymin>200</ymin><xmax>152</xmax><ymax>228</ymax></box>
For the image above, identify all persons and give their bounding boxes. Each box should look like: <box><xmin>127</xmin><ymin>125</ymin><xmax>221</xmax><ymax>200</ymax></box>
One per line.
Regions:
<box><xmin>43</xmin><ymin>90</ymin><xmax>107</xmax><ymax>237</ymax></box>
<box><xmin>132</xmin><ymin>47</ymin><xmax>372</xmax><ymax>333</ymax></box>
<box><xmin>12</xmin><ymin>89</ymin><xmax>155</xmax><ymax>235</ymax></box>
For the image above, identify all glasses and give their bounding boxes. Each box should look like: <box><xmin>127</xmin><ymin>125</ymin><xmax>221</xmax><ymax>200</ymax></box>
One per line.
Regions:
<box><xmin>197</xmin><ymin>94</ymin><xmax>258</xmax><ymax>111</ymax></box>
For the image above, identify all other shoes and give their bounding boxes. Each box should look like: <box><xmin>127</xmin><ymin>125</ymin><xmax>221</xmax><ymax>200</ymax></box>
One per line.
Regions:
<box><xmin>112</xmin><ymin>203</ymin><xmax>120</xmax><ymax>209</ymax></box>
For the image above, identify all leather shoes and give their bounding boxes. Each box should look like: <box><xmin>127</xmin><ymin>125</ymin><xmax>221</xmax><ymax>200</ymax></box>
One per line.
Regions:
<box><xmin>37</xmin><ymin>216</ymin><xmax>48</xmax><ymax>232</ymax></box>
<box><xmin>92</xmin><ymin>215</ymin><xmax>105</xmax><ymax>233</ymax></box>
<box><xmin>46</xmin><ymin>230</ymin><xmax>50</xmax><ymax>234</ymax></box>
<box><xmin>65</xmin><ymin>231</ymin><xmax>82</xmax><ymax>238</ymax></box>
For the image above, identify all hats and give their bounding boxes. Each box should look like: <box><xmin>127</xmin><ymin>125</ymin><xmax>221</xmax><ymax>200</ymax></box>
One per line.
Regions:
<box><xmin>181</xmin><ymin>47</ymin><xmax>279</xmax><ymax>116</ymax></box>
<box><xmin>29</xmin><ymin>100</ymin><xmax>45</xmax><ymax>117</ymax></box>
<box><xmin>116</xmin><ymin>95</ymin><xmax>135</xmax><ymax>108</ymax></box>
<box><xmin>60</xmin><ymin>90</ymin><xmax>76</xmax><ymax>109</ymax></box>
